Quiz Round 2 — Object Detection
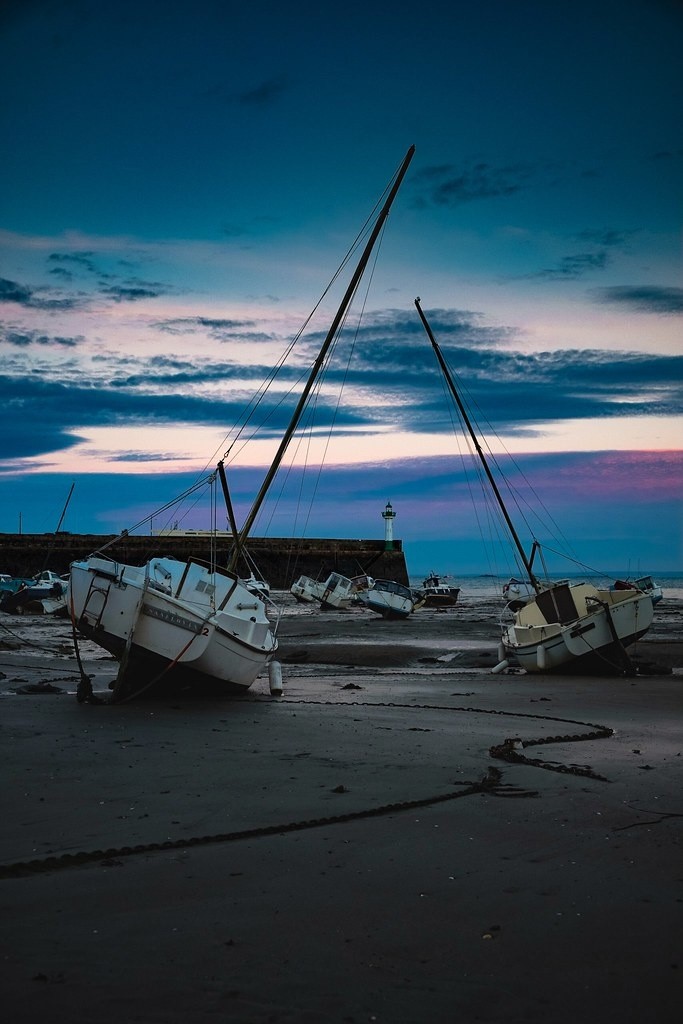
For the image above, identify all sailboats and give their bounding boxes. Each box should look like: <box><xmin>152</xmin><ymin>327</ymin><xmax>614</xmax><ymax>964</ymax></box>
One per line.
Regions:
<box><xmin>414</xmin><ymin>295</ymin><xmax>655</xmax><ymax>678</ymax></box>
<box><xmin>63</xmin><ymin>142</ymin><xmax>417</xmax><ymax>702</ymax></box>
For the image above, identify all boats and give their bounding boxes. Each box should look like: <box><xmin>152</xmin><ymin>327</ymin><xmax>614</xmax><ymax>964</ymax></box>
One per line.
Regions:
<box><xmin>502</xmin><ymin>577</ymin><xmax>573</xmax><ymax>614</ymax></box>
<box><xmin>349</xmin><ymin>575</ymin><xmax>375</xmax><ymax>606</ymax></box>
<box><xmin>290</xmin><ymin>574</ymin><xmax>317</xmax><ymax>604</ymax></box>
<box><xmin>311</xmin><ymin>571</ymin><xmax>357</xmax><ymax>612</ymax></box>
<box><xmin>608</xmin><ymin>576</ymin><xmax>663</xmax><ymax>606</ymax></box>
<box><xmin>0</xmin><ymin>570</ymin><xmax>69</xmax><ymax>618</ymax></box>
<box><xmin>419</xmin><ymin>570</ymin><xmax>460</xmax><ymax>607</ymax></box>
<box><xmin>357</xmin><ymin>578</ymin><xmax>415</xmax><ymax>619</ymax></box>
